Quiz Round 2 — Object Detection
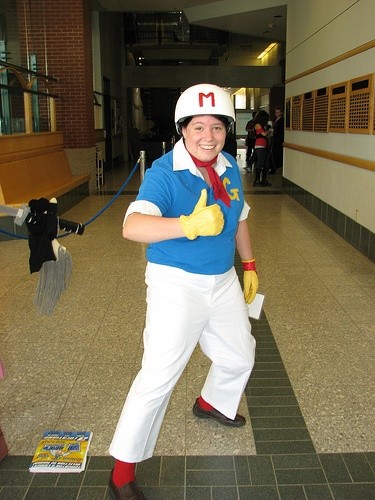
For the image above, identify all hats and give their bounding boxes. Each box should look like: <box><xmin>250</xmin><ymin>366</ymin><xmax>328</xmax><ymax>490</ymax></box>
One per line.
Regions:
<box><xmin>175</xmin><ymin>83</ymin><xmax>237</xmax><ymax>136</ymax></box>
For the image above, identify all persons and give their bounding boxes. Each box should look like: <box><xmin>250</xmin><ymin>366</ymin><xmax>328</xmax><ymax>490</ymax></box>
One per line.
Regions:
<box><xmin>267</xmin><ymin>107</ymin><xmax>284</xmax><ymax>175</ymax></box>
<box><xmin>109</xmin><ymin>83</ymin><xmax>258</xmax><ymax>500</ymax></box>
<box><xmin>243</xmin><ymin>111</ymin><xmax>257</xmax><ymax>173</ymax></box>
<box><xmin>255</xmin><ymin>110</ymin><xmax>272</xmax><ymax>187</ymax></box>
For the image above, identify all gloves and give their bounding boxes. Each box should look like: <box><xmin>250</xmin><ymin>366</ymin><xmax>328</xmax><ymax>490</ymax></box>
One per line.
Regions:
<box><xmin>179</xmin><ymin>189</ymin><xmax>225</xmax><ymax>240</ymax></box>
<box><xmin>242</xmin><ymin>257</ymin><xmax>259</xmax><ymax>304</ymax></box>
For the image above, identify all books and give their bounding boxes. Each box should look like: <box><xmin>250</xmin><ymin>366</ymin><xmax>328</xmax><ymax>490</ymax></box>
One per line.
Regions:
<box><xmin>28</xmin><ymin>429</ymin><xmax>93</xmax><ymax>475</ymax></box>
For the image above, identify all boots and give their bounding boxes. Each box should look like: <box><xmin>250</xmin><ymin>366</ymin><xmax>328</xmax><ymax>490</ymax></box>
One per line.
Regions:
<box><xmin>260</xmin><ymin>167</ymin><xmax>272</xmax><ymax>186</ymax></box>
<box><xmin>253</xmin><ymin>167</ymin><xmax>266</xmax><ymax>187</ymax></box>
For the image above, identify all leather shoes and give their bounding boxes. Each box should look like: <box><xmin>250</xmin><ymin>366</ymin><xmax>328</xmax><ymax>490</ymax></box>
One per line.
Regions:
<box><xmin>109</xmin><ymin>468</ymin><xmax>147</xmax><ymax>500</ymax></box>
<box><xmin>192</xmin><ymin>397</ymin><xmax>246</xmax><ymax>427</ymax></box>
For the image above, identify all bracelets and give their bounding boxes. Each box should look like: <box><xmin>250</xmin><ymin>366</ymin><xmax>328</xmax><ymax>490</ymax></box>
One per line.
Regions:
<box><xmin>267</xmin><ymin>129</ymin><xmax>271</xmax><ymax>134</ymax></box>
